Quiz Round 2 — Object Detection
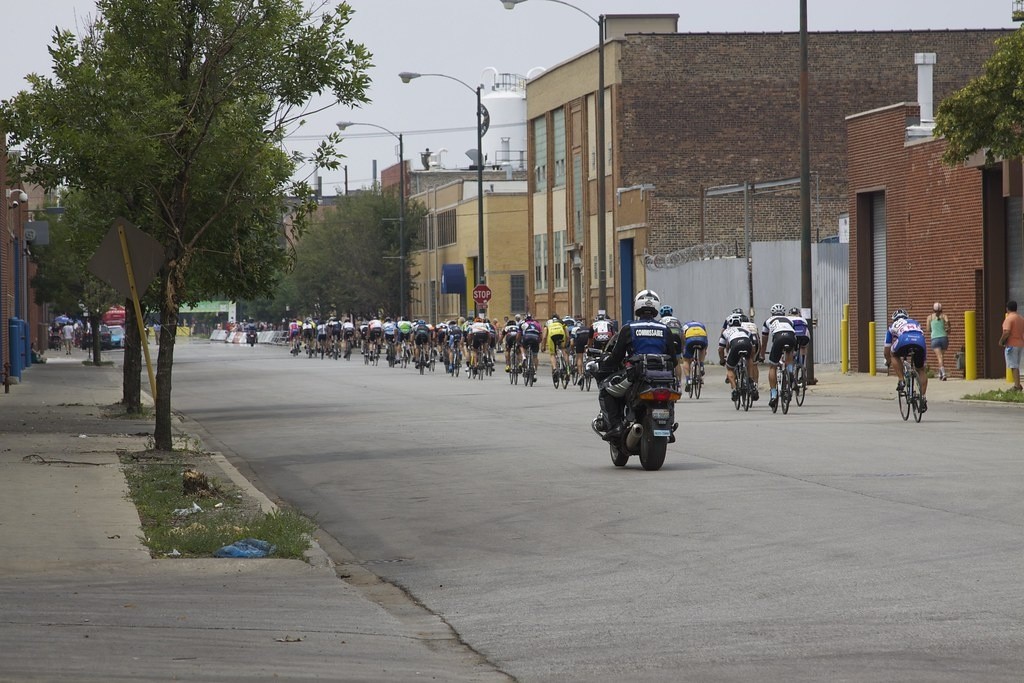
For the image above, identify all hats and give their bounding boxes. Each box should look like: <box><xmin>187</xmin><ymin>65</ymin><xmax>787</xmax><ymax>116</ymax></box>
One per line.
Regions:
<box><xmin>933</xmin><ymin>302</ymin><xmax>943</xmax><ymax>311</ymax></box>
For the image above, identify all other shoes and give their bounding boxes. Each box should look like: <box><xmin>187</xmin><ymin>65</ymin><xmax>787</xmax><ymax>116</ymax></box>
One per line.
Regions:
<box><xmin>505</xmin><ymin>365</ymin><xmax>510</xmax><ymax>372</ymax></box>
<box><xmin>769</xmin><ymin>399</ymin><xmax>776</xmax><ymax>408</ymax></box>
<box><xmin>938</xmin><ymin>375</ymin><xmax>943</xmax><ymax>379</ymax></box>
<box><xmin>577</xmin><ymin>376</ymin><xmax>583</xmax><ymax>385</ymax></box>
<box><xmin>941</xmin><ymin>375</ymin><xmax>947</xmax><ymax>381</ymax></box>
<box><xmin>752</xmin><ymin>390</ymin><xmax>759</xmax><ymax>400</ymax></box>
<box><xmin>685</xmin><ymin>385</ymin><xmax>690</xmax><ymax>392</ymax></box>
<box><xmin>552</xmin><ymin>369</ymin><xmax>559</xmax><ymax>375</ymax></box>
<box><xmin>895</xmin><ymin>380</ymin><xmax>906</xmax><ymax>390</ymax></box>
<box><xmin>522</xmin><ymin>357</ymin><xmax>526</xmax><ymax>366</ymax></box>
<box><xmin>701</xmin><ymin>371</ymin><xmax>705</xmax><ymax>377</ymax></box>
<box><xmin>919</xmin><ymin>400</ymin><xmax>927</xmax><ymax>413</ymax></box>
<box><xmin>532</xmin><ymin>375</ymin><xmax>538</xmax><ymax>382</ymax></box>
<box><xmin>1006</xmin><ymin>386</ymin><xmax>1022</xmax><ymax>392</ymax></box>
<box><xmin>731</xmin><ymin>390</ymin><xmax>741</xmax><ymax>402</ymax></box>
<box><xmin>518</xmin><ymin>363</ymin><xmax>523</xmax><ymax>372</ymax></box>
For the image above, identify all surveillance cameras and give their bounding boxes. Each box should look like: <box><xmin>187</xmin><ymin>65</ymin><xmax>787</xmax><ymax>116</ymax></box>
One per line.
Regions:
<box><xmin>19</xmin><ymin>193</ymin><xmax>28</xmax><ymax>203</ymax></box>
<box><xmin>12</xmin><ymin>201</ymin><xmax>19</xmax><ymax>208</ymax></box>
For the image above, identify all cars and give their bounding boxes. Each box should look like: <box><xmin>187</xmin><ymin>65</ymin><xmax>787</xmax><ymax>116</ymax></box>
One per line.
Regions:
<box><xmin>82</xmin><ymin>325</ymin><xmax>125</xmax><ymax>349</ymax></box>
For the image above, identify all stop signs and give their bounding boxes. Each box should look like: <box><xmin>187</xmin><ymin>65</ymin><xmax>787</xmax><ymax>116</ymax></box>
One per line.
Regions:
<box><xmin>472</xmin><ymin>284</ymin><xmax>492</xmax><ymax>305</ymax></box>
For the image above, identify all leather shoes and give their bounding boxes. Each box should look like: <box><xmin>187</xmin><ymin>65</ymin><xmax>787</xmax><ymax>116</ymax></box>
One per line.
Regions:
<box><xmin>602</xmin><ymin>425</ymin><xmax>624</xmax><ymax>440</ymax></box>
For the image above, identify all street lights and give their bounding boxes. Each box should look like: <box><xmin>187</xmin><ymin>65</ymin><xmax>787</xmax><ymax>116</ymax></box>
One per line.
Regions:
<box><xmin>499</xmin><ymin>0</ymin><xmax>609</xmax><ymax>319</ymax></box>
<box><xmin>400</xmin><ymin>68</ymin><xmax>488</xmax><ymax>319</ymax></box>
<box><xmin>337</xmin><ymin>120</ymin><xmax>406</xmax><ymax>318</ymax></box>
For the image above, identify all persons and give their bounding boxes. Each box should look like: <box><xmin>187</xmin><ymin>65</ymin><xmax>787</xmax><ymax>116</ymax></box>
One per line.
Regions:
<box><xmin>759</xmin><ymin>303</ymin><xmax>797</xmax><ymax>407</ymax></box>
<box><xmin>681</xmin><ymin>320</ymin><xmax>708</xmax><ymax>392</ymax></box>
<box><xmin>717</xmin><ymin>308</ymin><xmax>762</xmax><ymax>401</ymax></box>
<box><xmin>785</xmin><ymin>307</ymin><xmax>811</xmax><ymax>388</ymax></box>
<box><xmin>62</xmin><ymin>322</ymin><xmax>74</xmax><ymax>355</ymax></box>
<box><xmin>288</xmin><ymin>313</ymin><xmax>617</xmax><ymax>385</ymax></box>
<box><xmin>927</xmin><ymin>303</ymin><xmax>951</xmax><ymax>381</ymax></box>
<box><xmin>142</xmin><ymin>324</ymin><xmax>160</xmax><ymax>345</ymax></box>
<box><xmin>884</xmin><ymin>309</ymin><xmax>928</xmax><ymax>414</ymax></box>
<box><xmin>658</xmin><ymin>305</ymin><xmax>684</xmax><ymax>400</ymax></box>
<box><xmin>999</xmin><ymin>301</ymin><xmax>1024</xmax><ymax>393</ymax></box>
<box><xmin>594</xmin><ymin>289</ymin><xmax>679</xmax><ymax>440</ymax></box>
<box><xmin>48</xmin><ymin>322</ymin><xmax>82</xmax><ymax>347</ymax></box>
<box><xmin>224</xmin><ymin>317</ymin><xmax>283</xmax><ymax>344</ymax></box>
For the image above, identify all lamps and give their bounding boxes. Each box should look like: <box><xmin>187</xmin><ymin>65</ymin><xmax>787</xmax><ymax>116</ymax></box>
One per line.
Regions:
<box><xmin>6</xmin><ymin>188</ymin><xmax>29</xmax><ymax>203</ymax></box>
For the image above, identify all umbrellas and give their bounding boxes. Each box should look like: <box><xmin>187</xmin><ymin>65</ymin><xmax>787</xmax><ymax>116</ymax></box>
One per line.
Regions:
<box><xmin>50</xmin><ymin>314</ymin><xmax>84</xmax><ymax>323</ymax></box>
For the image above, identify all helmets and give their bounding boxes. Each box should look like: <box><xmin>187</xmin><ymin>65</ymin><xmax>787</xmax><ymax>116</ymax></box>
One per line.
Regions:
<box><xmin>595</xmin><ymin>314</ymin><xmax>604</xmax><ymax>321</ymax></box>
<box><xmin>729</xmin><ymin>316</ymin><xmax>742</xmax><ymax>326</ymax></box>
<box><xmin>660</xmin><ymin>305</ymin><xmax>673</xmax><ymax>317</ymax></box>
<box><xmin>574</xmin><ymin>320</ymin><xmax>586</xmax><ymax>327</ymax></box>
<box><xmin>789</xmin><ymin>307</ymin><xmax>801</xmax><ymax>314</ymax></box>
<box><xmin>771</xmin><ymin>304</ymin><xmax>786</xmax><ymax>315</ymax></box>
<box><xmin>733</xmin><ymin>308</ymin><xmax>743</xmax><ymax>313</ymax></box>
<box><xmin>892</xmin><ymin>308</ymin><xmax>909</xmax><ymax>321</ymax></box>
<box><xmin>291</xmin><ymin>314</ymin><xmax>559</xmax><ymax>325</ymax></box>
<box><xmin>634</xmin><ymin>289</ymin><xmax>660</xmax><ymax>317</ymax></box>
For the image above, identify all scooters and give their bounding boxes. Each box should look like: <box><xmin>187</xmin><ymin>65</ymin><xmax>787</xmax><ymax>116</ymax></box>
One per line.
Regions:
<box><xmin>583</xmin><ymin>333</ymin><xmax>683</xmax><ymax>471</ymax></box>
<box><xmin>245</xmin><ymin>330</ymin><xmax>258</xmax><ymax>347</ymax></box>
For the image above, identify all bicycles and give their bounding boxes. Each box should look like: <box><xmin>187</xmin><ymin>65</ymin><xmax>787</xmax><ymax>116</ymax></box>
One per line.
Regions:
<box><xmin>672</xmin><ymin>341</ymin><xmax>683</xmax><ymax>401</ymax></box>
<box><xmin>687</xmin><ymin>345</ymin><xmax>705</xmax><ymax>399</ymax></box>
<box><xmin>885</xmin><ymin>347</ymin><xmax>926</xmax><ymax>422</ymax></box>
<box><xmin>783</xmin><ymin>340</ymin><xmax>810</xmax><ymax>408</ymax></box>
<box><xmin>722</xmin><ymin>350</ymin><xmax>755</xmax><ymax>411</ymax></box>
<box><xmin>761</xmin><ymin>343</ymin><xmax>795</xmax><ymax>414</ymax></box>
<box><xmin>288</xmin><ymin>330</ymin><xmax>595</xmax><ymax>392</ymax></box>
